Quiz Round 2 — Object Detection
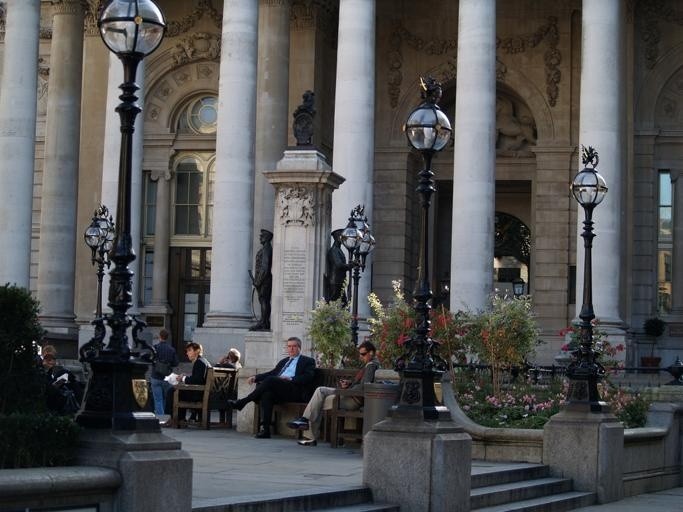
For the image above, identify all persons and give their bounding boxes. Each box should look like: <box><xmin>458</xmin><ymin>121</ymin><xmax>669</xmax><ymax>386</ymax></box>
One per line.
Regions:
<box><xmin>38</xmin><ymin>344</ymin><xmax>83</xmax><ymax>414</ymax></box>
<box><xmin>157</xmin><ymin>340</ymin><xmax>216</xmax><ymax>428</ymax></box>
<box><xmin>246</xmin><ymin>228</ymin><xmax>273</xmax><ymax>332</ymax></box>
<box><xmin>147</xmin><ymin>329</ymin><xmax>179</xmax><ymax>416</ymax></box>
<box><xmin>227</xmin><ymin>336</ymin><xmax>316</xmax><ymax>438</ymax></box>
<box><xmin>326</xmin><ymin>228</ymin><xmax>360</xmax><ymax>308</ymax></box>
<box><xmin>285</xmin><ymin>340</ymin><xmax>379</xmax><ymax>446</ymax></box>
<box><xmin>188</xmin><ymin>346</ymin><xmax>241</xmax><ymax>423</ymax></box>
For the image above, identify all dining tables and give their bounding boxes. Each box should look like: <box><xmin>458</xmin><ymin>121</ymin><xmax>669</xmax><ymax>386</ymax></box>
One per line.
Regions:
<box><xmin>640</xmin><ymin>317</ymin><xmax>668</xmax><ymax>373</ymax></box>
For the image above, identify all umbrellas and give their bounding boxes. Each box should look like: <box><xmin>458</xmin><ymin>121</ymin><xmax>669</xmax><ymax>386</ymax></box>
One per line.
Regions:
<box><xmin>256</xmin><ymin>429</ymin><xmax>270</xmax><ymax>437</ymax></box>
<box><xmin>228</xmin><ymin>398</ymin><xmax>244</xmax><ymax>411</ymax></box>
<box><xmin>159</xmin><ymin>418</ymin><xmax>174</xmax><ymax>426</ymax></box>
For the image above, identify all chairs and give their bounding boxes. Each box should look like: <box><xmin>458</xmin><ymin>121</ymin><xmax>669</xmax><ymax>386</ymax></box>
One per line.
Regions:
<box><xmin>287</xmin><ymin>417</ymin><xmax>308</xmax><ymax>430</ymax></box>
<box><xmin>298</xmin><ymin>437</ymin><xmax>317</xmax><ymax>446</ymax></box>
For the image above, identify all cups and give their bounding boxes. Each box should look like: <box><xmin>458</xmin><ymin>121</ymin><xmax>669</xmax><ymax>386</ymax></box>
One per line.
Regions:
<box><xmin>360</xmin><ymin>351</ymin><xmax>368</xmax><ymax>356</ymax></box>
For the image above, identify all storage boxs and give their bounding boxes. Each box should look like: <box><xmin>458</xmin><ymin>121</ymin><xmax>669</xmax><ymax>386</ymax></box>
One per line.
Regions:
<box><xmin>172</xmin><ymin>368</ymin><xmax>239</xmax><ymax>430</ymax></box>
<box><xmin>252</xmin><ymin>369</ymin><xmax>370</xmax><ymax>449</ymax></box>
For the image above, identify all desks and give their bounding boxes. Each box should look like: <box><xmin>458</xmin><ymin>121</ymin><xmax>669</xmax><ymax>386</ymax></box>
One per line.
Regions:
<box><xmin>66</xmin><ymin>1</ymin><xmax>168</xmax><ymax>440</ymax></box>
<box><xmin>558</xmin><ymin>143</ymin><xmax>615</xmax><ymax>413</ymax></box>
<box><xmin>61</xmin><ymin>201</ymin><xmax>124</xmax><ymax>430</ymax></box>
<box><xmin>336</xmin><ymin>199</ymin><xmax>378</xmax><ymax>354</ymax></box>
<box><xmin>385</xmin><ymin>73</ymin><xmax>454</xmax><ymax>424</ymax></box>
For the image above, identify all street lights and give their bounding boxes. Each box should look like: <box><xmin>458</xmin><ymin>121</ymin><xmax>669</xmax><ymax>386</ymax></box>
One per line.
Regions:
<box><xmin>154</xmin><ymin>360</ymin><xmax>171</xmax><ymax>376</ymax></box>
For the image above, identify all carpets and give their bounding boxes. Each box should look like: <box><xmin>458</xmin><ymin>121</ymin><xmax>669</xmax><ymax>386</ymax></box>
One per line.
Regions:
<box><xmin>279</xmin><ymin>358</ymin><xmax>293</xmax><ymax>376</ymax></box>
